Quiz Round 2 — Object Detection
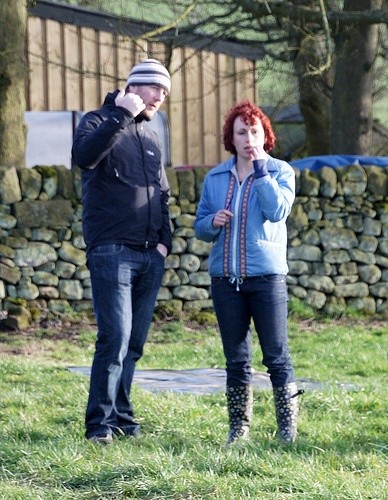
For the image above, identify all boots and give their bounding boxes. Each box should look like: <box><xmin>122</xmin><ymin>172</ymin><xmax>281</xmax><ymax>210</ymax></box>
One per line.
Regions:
<box><xmin>226</xmin><ymin>384</ymin><xmax>254</xmax><ymax>443</ymax></box>
<box><xmin>273</xmin><ymin>383</ymin><xmax>298</xmax><ymax>442</ymax></box>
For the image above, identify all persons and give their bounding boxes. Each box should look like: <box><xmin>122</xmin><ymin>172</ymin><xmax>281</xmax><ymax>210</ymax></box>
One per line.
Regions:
<box><xmin>71</xmin><ymin>58</ymin><xmax>175</xmax><ymax>445</ymax></box>
<box><xmin>194</xmin><ymin>99</ymin><xmax>297</xmax><ymax>445</ymax></box>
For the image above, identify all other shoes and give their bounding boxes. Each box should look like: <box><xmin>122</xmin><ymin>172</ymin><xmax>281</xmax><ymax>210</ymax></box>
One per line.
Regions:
<box><xmin>93</xmin><ymin>433</ymin><xmax>112</xmax><ymax>443</ymax></box>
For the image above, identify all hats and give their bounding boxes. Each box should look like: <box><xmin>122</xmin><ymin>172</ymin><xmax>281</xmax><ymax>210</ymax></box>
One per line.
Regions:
<box><xmin>125</xmin><ymin>59</ymin><xmax>171</xmax><ymax>93</ymax></box>
